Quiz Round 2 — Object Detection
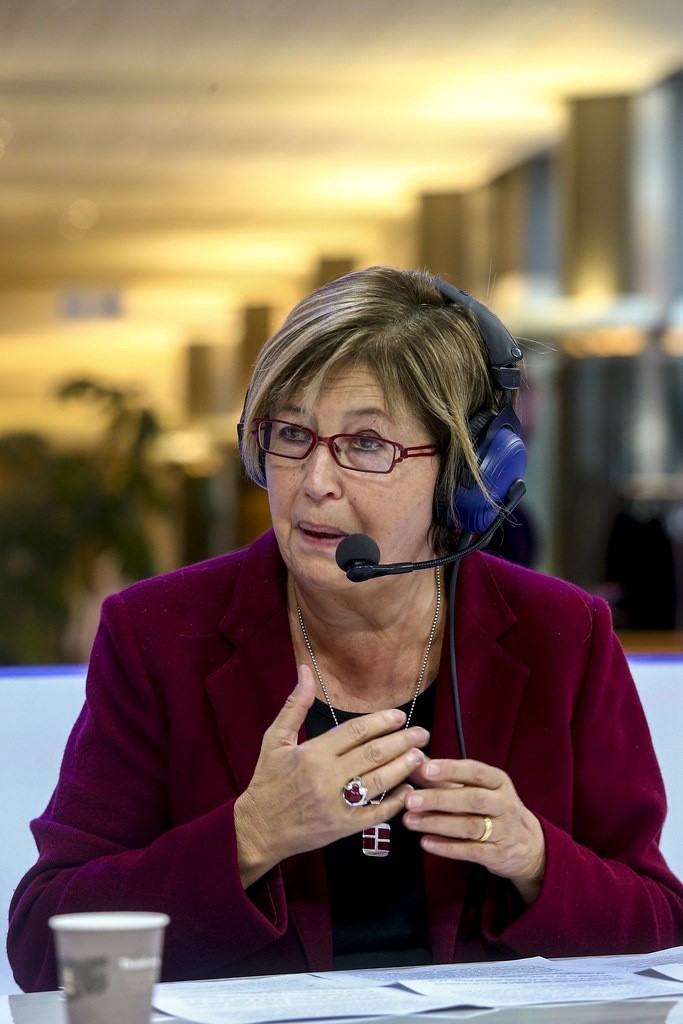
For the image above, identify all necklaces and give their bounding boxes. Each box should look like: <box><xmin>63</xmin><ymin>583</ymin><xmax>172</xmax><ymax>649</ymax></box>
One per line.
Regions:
<box><xmin>293</xmin><ymin>563</ymin><xmax>442</xmax><ymax>858</ymax></box>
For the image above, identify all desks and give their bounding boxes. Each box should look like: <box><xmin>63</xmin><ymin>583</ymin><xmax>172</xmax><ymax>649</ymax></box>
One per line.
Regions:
<box><xmin>5</xmin><ymin>950</ymin><xmax>683</xmax><ymax>1024</ymax></box>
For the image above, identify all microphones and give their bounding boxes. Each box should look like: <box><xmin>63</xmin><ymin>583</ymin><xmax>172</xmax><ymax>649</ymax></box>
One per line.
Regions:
<box><xmin>335</xmin><ymin>478</ymin><xmax>526</xmax><ymax>582</ymax></box>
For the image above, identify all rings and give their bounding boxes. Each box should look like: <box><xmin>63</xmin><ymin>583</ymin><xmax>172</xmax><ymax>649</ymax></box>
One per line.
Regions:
<box><xmin>476</xmin><ymin>815</ymin><xmax>493</xmax><ymax>842</ymax></box>
<box><xmin>343</xmin><ymin>775</ymin><xmax>365</xmax><ymax>806</ymax></box>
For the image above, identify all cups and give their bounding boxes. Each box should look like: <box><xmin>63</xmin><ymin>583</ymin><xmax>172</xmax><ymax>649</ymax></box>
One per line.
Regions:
<box><xmin>45</xmin><ymin>911</ymin><xmax>170</xmax><ymax>1024</ymax></box>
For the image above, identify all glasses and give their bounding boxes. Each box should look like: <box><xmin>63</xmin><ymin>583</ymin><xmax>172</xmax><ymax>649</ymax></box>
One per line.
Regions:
<box><xmin>239</xmin><ymin>416</ymin><xmax>445</xmax><ymax>474</ymax></box>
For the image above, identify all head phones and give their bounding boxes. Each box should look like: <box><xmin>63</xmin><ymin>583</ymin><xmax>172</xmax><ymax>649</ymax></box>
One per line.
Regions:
<box><xmin>236</xmin><ymin>273</ymin><xmax>527</xmax><ymax>536</ymax></box>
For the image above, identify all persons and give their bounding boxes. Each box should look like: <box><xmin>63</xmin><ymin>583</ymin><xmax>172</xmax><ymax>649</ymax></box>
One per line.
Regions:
<box><xmin>7</xmin><ymin>265</ymin><xmax>683</xmax><ymax>993</ymax></box>
